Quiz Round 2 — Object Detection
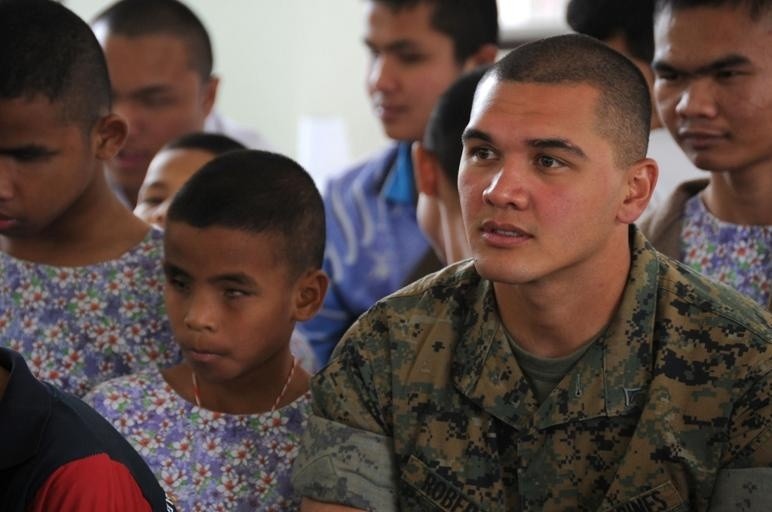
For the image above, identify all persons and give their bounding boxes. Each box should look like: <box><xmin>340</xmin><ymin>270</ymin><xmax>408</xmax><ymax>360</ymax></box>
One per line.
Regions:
<box><xmin>284</xmin><ymin>31</ymin><xmax>770</xmax><ymax>510</ymax></box>
<box><xmin>634</xmin><ymin>0</ymin><xmax>771</xmax><ymax>314</ymax></box>
<box><xmin>130</xmin><ymin>133</ymin><xmax>247</xmax><ymax>232</ymax></box>
<box><xmin>1</xmin><ymin>343</ymin><xmax>170</xmax><ymax>511</ymax></box>
<box><xmin>280</xmin><ymin>1</ymin><xmax>505</xmax><ymax>377</ymax></box>
<box><xmin>88</xmin><ymin>1</ymin><xmax>221</xmax><ymax>212</ymax></box>
<box><xmin>72</xmin><ymin>148</ymin><xmax>334</xmax><ymax>511</ymax></box>
<box><xmin>565</xmin><ymin>1</ymin><xmax>712</xmax><ymax>228</ymax></box>
<box><xmin>1</xmin><ymin>0</ymin><xmax>179</xmax><ymax>393</ymax></box>
<box><xmin>404</xmin><ymin>62</ymin><xmax>481</xmax><ymax>270</ymax></box>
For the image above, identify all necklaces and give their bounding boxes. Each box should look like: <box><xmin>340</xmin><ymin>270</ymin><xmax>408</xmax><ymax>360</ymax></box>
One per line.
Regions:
<box><xmin>183</xmin><ymin>354</ymin><xmax>297</xmax><ymax>414</ymax></box>
